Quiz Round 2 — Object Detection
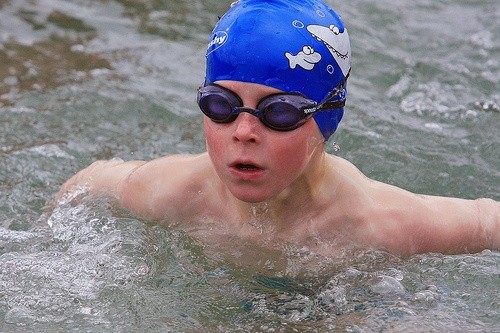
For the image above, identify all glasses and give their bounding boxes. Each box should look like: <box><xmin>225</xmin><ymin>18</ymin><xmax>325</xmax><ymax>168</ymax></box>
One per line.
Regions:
<box><xmin>197</xmin><ymin>82</ymin><xmax>348</xmax><ymax>132</ymax></box>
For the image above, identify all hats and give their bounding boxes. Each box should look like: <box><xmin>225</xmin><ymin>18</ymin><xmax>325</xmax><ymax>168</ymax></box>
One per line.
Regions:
<box><xmin>203</xmin><ymin>0</ymin><xmax>350</xmax><ymax>140</ymax></box>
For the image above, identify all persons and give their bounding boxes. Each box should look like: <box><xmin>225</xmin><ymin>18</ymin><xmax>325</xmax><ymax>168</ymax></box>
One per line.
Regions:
<box><xmin>39</xmin><ymin>0</ymin><xmax>500</xmax><ymax>280</ymax></box>
